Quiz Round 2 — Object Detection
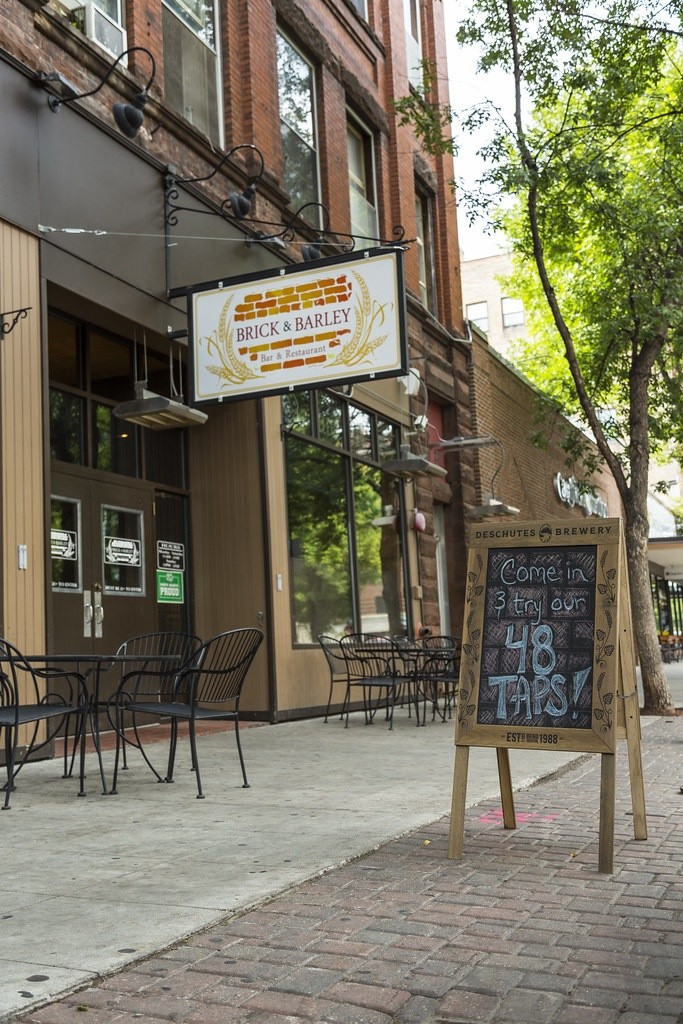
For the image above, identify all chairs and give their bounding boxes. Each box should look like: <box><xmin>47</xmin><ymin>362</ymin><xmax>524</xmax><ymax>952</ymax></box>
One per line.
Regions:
<box><xmin>0</xmin><ymin>628</ymin><xmax>265</xmax><ymax>810</ymax></box>
<box><xmin>318</xmin><ymin>634</ymin><xmax>462</xmax><ymax>730</ymax></box>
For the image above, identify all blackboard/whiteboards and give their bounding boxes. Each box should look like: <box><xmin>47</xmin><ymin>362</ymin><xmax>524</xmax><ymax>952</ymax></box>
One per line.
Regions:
<box><xmin>455</xmin><ymin>516</ymin><xmax>622</xmax><ymax>752</ymax></box>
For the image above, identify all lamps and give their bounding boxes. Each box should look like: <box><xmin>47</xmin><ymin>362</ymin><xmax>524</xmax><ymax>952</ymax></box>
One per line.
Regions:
<box><xmin>114</xmin><ymin>328</ymin><xmax>208</xmax><ymax>429</ymax></box>
<box><xmin>163</xmin><ymin>145</ymin><xmax>264</xmax><ymax>220</ymax></box>
<box><xmin>48</xmin><ymin>47</ymin><xmax>156</xmax><ymax>138</ymax></box>
<box><xmin>381</xmin><ymin>370</ymin><xmax>447</xmax><ymax>481</ymax></box>
<box><xmin>471</xmin><ymin>444</ymin><xmax>520</xmax><ymax>517</ymax></box>
<box><xmin>397</xmin><ymin>368</ymin><xmax>420</xmax><ymax>395</ymax></box>
<box><xmin>246</xmin><ymin>202</ymin><xmax>330</xmax><ymax>263</ymax></box>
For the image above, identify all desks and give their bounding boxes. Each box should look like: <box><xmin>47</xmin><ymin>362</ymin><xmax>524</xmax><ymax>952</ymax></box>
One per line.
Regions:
<box><xmin>355</xmin><ymin>648</ymin><xmax>455</xmax><ymax>726</ymax></box>
<box><xmin>0</xmin><ymin>655</ymin><xmax>182</xmax><ymax>797</ymax></box>
